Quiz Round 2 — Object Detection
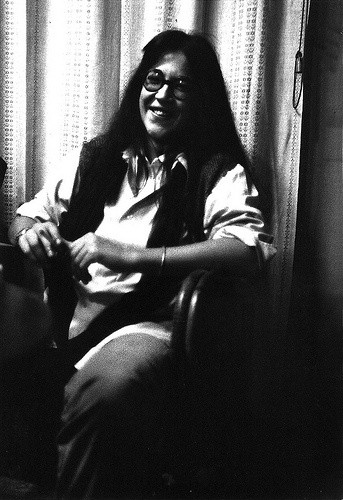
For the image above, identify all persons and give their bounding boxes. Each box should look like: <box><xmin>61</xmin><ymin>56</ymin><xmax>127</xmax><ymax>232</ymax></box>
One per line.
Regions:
<box><xmin>8</xmin><ymin>28</ymin><xmax>277</xmax><ymax>497</ymax></box>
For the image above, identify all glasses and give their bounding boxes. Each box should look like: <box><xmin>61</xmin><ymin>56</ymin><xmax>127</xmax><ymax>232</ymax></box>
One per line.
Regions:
<box><xmin>142</xmin><ymin>69</ymin><xmax>195</xmax><ymax>101</ymax></box>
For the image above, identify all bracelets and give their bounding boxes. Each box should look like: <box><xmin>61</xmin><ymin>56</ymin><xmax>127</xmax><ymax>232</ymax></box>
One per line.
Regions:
<box><xmin>158</xmin><ymin>245</ymin><xmax>166</xmax><ymax>278</ymax></box>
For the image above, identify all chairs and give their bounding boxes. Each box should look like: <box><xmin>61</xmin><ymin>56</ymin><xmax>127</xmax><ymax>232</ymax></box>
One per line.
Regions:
<box><xmin>0</xmin><ymin>164</ymin><xmax>272</xmax><ymax>500</ymax></box>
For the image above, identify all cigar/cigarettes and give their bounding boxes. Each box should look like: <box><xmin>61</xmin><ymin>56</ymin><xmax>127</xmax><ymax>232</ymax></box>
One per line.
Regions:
<box><xmin>39</xmin><ymin>230</ymin><xmax>59</xmax><ymax>250</ymax></box>
